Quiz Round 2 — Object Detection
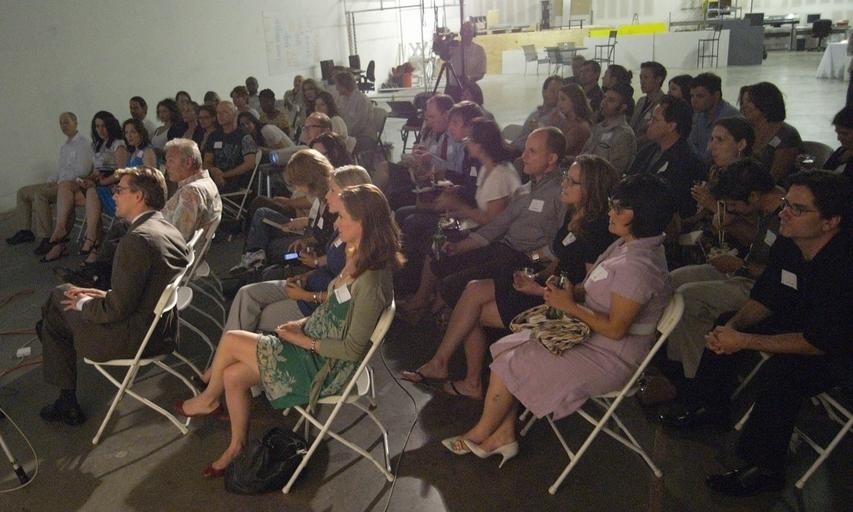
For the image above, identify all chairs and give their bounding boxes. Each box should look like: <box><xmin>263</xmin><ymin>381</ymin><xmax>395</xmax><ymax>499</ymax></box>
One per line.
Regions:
<box><xmin>219</xmin><ymin>150</ymin><xmax>262</xmax><ymax>242</ymax></box>
<box><xmin>697</xmin><ymin>23</ymin><xmax>723</xmax><ymax>69</ymax></box>
<box><xmin>281</xmin><ymin>287</ymin><xmax>395</xmax><ymax>496</ymax></box>
<box><xmin>735</xmin><ymin>391</ymin><xmax>852</xmax><ymax>490</ymax></box>
<box><xmin>520</xmin><ymin>292</ymin><xmax>686</xmax><ymax>496</ymax></box>
<box><xmin>808</xmin><ymin>19</ymin><xmax>832</xmax><ymax>52</ymax></box>
<box><xmin>170</xmin><ymin>228</ymin><xmax>214</xmax><ymax>378</ymax></box>
<box><xmin>84</xmin><ymin>250</ymin><xmax>200</xmax><ymax>445</ymax></box>
<box><xmin>177</xmin><ymin>213</ymin><xmax>227</xmax><ymax>333</ymax></box>
<box><xmin>194</xmin><ymin>261</ymin><xmax>227</xmax><ymax>303</ymax></box>
<box><xmin>521</xmin><ymin>30</ymin><xmax>618</xmax><ymax>78</ymax></box>
<box><xmin>320</xmin><ymin>54</ymin><xmax>375</xmax><ymax>94</ymax></box>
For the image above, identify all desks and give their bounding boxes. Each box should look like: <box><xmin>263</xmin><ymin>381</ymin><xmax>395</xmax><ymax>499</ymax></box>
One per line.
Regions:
<box><xmin>796</xmin><ymin>26</ymin><xmax>851</xmax><ymax>51</ymax></box>
<box><xmin>763</xmin><ymin>18</ymin><xmax>799</xmax><ymax>51</ymax></box>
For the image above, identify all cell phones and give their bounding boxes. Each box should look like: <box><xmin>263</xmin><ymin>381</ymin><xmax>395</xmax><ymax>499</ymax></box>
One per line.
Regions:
<box><xmin>284</xmin><ymin>252</ymin><xmax>299</xmax><ymax>263</ymax></box>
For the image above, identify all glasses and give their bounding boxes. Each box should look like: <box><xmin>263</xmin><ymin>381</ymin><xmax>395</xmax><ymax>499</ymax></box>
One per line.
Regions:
<box><xmin>561</xmin><ymin>168</ymin><xmax>581</xmax><ymax>189</ymax></box>
<box><xmin>606</xmin><ymin>197</ymin><xmax>634</xmax><ymax>216</ymax></box>
<box><xmin>778</xmin><ymin>196</ymin><xmax>821</xmax><ymax>218</ymax></box>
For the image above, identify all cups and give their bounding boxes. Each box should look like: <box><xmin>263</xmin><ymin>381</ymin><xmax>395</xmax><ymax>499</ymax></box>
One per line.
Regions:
<box><xmin>301</xmin><ymin>246</ymin><xmax>315</xmax><ymax>256</ymax></box>
<box><xmin>690</xmin><ymin>179</ymin><xmax>708</xmax><ymax>192</ymax></box>
<box><xmin>517</xmin><ymin>267</ymin><xmax>535</xmax><ymax>278</ymax></box>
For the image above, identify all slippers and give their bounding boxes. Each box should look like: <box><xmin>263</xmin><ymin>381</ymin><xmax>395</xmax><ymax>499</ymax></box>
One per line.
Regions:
<box><xmin>437</xmin><ymin>380</ymin><xmax>484</xmax><ymax>400</ymax></box>
<box><xmin>399</xmin><ymin>370</ymin><xmax>448</xmax><ymax>384</ymax></box>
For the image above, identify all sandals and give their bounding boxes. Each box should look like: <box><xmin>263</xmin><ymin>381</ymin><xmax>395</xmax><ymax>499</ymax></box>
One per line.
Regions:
<box><xmin>5</xmin><ymin>230</ymin><xmax>100</xmax><ymax>269</ymax></box>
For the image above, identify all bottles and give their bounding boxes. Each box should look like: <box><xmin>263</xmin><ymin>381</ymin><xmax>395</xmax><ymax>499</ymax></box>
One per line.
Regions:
<box><xmin>548</xmin><ymin>270</ymin><xmax>571</xmax><ymax>321</ymax></box>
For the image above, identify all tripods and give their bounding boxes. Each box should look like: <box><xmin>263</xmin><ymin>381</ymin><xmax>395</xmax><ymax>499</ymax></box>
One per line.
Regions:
<box><xmin>432</xmin><ymin>61</ymin><xmax>465</xmax><ymax>96</ymax></box>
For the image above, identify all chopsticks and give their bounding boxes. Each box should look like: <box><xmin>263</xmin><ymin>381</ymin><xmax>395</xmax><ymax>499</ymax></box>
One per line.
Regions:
<box><xmin>717</xmin><ymin>199</ymin><xmax>728</xmax><ymax>247</ymax></box>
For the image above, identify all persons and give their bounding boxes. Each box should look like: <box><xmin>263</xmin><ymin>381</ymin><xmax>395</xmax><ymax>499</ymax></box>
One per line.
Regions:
<box><xmin>634</xmin><ymin>155</ymin><xmax>788</xmax><ymax>430</ymax></box>
<box><xmin>3</xmin><ymin>20</ymin><xmax>853</xmax><ymax>407</ymax></box>
<box><xmin>658</xmin><ymin>169</ymin><xmax>853</xmax><ymax>499</ymax></box>
<box><xmin>35</xmin><ymin>165</ymin><xmax>193</xmax><ymax>426</ymax></box>
<box><xmin>174</xmin><ymin>181</ymin><xmax>402</xmax><ymax>482</ymax></box>
<box><xmin>439</xmin><ymin>169</ymin><xmax>680</xmax><ymax>469</ymax></box>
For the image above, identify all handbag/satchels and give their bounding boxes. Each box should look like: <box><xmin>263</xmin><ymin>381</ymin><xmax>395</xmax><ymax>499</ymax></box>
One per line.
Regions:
<box><xmin>224</xmin><ymin>427</ymin><xmax>308</xmax><ymax>495</ymax></box>
<box><xmin>526</xmin><ymin>303</ymin><xmax>592</xmax><ymax>357</ymax></box>
<box><xmin>76</xmin><ymin>175</ymin><xmax>96</xmax><ymax>189</ymax></box>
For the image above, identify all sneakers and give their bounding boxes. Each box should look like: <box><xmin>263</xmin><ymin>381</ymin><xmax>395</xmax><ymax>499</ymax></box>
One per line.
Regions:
<box><xmin>228</xmin><ymin>249</ymin><xmax>267</xmax><ymax>279</ymax></box>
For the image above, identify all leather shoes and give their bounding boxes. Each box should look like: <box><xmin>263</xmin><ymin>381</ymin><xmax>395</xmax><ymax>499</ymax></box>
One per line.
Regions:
<box><xmin>658</xmin><ymin>405</ymin><xmax>731</xmax><ymax>428</ymax></box>
<box><xmin>36</xmin><ymin>319</ymin><xmax>43</xmax><ymax>343</ymax></box>
<box><xmin>170</xmin><ymin>375</ymin><xmax>248</xmax><ymax>481</ymax></box>
<box><xmin>705</xmin><ymin>461</ymin><xmax>786</xmax><ymax>497</ymax></box>
<box><xmin>40</xmin><ymin>404</ymin><xmax>86</xmax><ymax>425</ymax></box>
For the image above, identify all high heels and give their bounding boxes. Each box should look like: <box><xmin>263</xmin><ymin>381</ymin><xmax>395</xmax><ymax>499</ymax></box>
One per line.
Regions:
<box><xmin>393</xmin><ymin>301</ymin><xmax>430</xmax><ymax>327</ymax></box>
<box><xmin>440</xmin><ymin>435</ymin><xmax>520</xmax><ymax>469</ymax></box>
